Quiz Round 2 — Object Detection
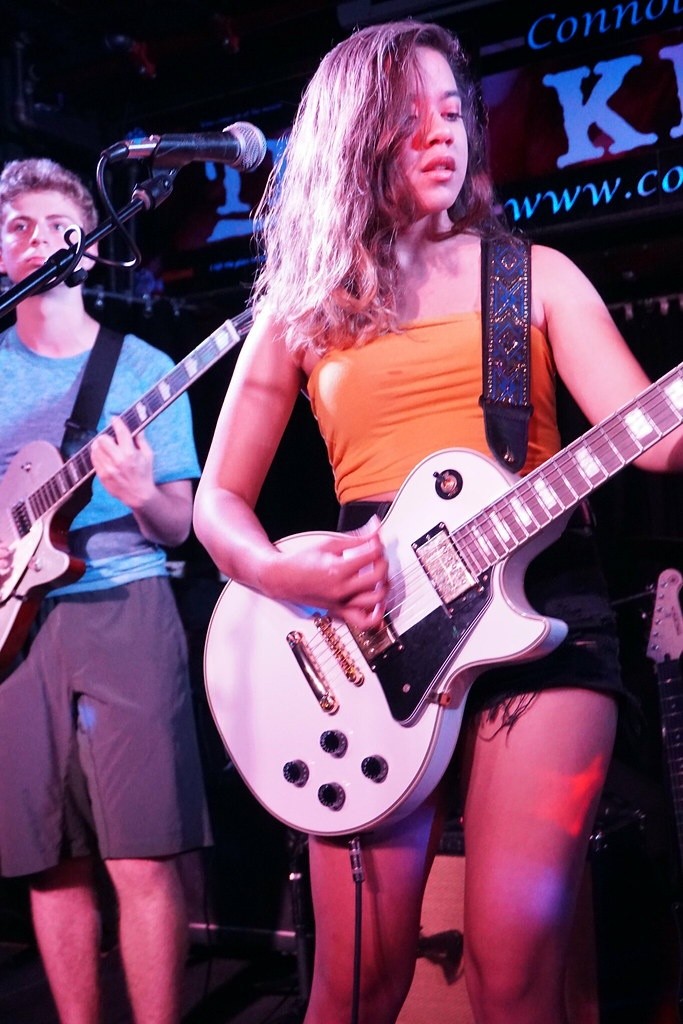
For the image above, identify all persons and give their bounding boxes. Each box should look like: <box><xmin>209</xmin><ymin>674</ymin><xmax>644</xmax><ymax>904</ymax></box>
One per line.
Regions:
<box><xmin>0</xmin><ymin>159</ymin><xmax>213</xmax><ymax>1024</ymax></box>
<box><xmin>193</xmin><ymin>22</ymin><xmax>683</xmax><ymax>1024</ymax></box>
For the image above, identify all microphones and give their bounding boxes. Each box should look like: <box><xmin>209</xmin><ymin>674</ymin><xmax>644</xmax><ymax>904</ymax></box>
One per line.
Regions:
<box><xmin>116</xmin><ymin>121</ymin><xmax>271</xmax><ymax>172</ymax></box>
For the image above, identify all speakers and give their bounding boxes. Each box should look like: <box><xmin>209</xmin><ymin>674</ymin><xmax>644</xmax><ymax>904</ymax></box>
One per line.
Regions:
<box><xmin>188</xmin><ymin>633</ymin><xmax>313</xmax><ymax>957</ymax></box>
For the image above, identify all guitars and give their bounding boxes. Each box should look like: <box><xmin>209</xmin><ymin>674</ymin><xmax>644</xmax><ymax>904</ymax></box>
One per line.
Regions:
<box><xmin>201</xmin><ymin>360</ymin><xmax>683</xmax><ymax>838</ymax></box>
<box><xmin>647</xmin><ymin>567</ymin><xmax>683</xmax><ymax>808</ymax></box>
<box><xmin>0</xmin><ymin>314</ymin><xmax>241</xmax><ymax>656</ymax></box>
